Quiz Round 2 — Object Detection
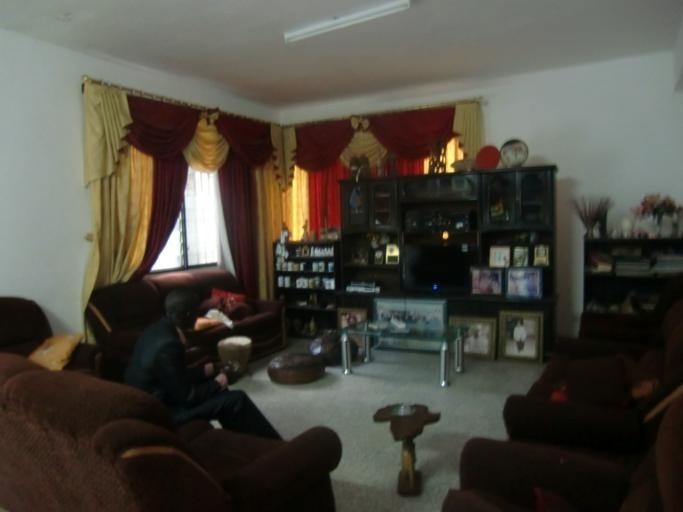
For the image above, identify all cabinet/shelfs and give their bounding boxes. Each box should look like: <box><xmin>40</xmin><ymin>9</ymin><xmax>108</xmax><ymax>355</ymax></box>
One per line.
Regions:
<box><xmin>336</xmin><ymin>165</ymin><xmax>556</xmax><ymax>352</ymax></box>
<box><xmin>583</xmin><ymin>237</ymin><xmax>681</xmax><ymax>312</ymax></box>
<box><xmin>272</xmin><ymin>239</ymin><xmax>339</xmax><ymax>341</ymax></box>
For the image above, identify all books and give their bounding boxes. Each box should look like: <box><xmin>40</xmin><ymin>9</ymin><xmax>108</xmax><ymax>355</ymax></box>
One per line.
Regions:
<box><xmin>345</xmin><ymin>280</ymin><xmax>383</xmax><ymax>295</ymax></box>
<box><xmin>588</xmin><ymin>243</ymin><xmax>683</xmax><ymax>275</ymax></box>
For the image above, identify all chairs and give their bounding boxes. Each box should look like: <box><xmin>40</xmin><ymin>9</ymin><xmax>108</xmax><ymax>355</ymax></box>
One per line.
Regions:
<box><xmin>443</xmin><ymin>294</ymin><xmax>683</xmax><ymax>512</ymax></box>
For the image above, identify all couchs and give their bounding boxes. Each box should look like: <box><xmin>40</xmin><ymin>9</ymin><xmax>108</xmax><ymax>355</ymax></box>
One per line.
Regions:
<box><xmin>1</xmin><ymin>269</ymin><xmax>345</xmax><ymax>511</ymax></box>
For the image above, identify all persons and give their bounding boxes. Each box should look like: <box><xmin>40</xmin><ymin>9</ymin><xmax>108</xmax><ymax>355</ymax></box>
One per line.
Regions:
<box><xmin>123</xmin><ymin>288</ymin><xmax>286</xmax><ymax>443</ymax></box>
<box><xmin>514</xmin><ymin>319</ymin><xmax>525</xmax><ymax>352</ymax></box>
